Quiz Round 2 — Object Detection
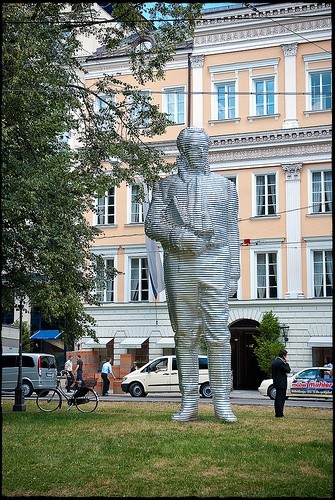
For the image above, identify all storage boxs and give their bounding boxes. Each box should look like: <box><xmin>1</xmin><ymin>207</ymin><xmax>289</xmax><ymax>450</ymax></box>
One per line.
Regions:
<box><xmin>65</xmin><ymin>351</ymin><xmax>136</xmax><ymax>394</ymax></box>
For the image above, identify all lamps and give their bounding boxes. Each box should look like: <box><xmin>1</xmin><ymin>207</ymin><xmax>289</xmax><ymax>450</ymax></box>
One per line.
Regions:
<box><xmin>280</xmin><ymin>324</ymin><xmax>290</xmax><ymax>341</ymax></box>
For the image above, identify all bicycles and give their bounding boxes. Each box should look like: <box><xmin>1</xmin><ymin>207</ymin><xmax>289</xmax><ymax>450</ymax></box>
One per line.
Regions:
<box><xmin>37</xmin><ymin>370</ymin><xmax>99</xmax><ymax>413</ymax></box>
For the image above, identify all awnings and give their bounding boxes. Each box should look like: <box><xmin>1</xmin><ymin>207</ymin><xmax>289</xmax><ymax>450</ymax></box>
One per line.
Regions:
<box><xmin>28</xmin><ymin>330</ymin><xmax>65</xmax><ymax>339</ymax></box>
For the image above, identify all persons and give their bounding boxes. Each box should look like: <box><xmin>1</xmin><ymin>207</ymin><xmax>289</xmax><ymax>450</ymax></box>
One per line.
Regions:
<box><xmin>272</xmin><ymin>349</ymin><xmax>291</xmax><ymax>418</ymax></box>
<box><xmin>101</xmin><ymin>357</ymin><xmax>116</xmax><ymax>397</ymax></box>
<box><xmin>130</xmin><ymin>361</ymin><xmax>141</xmax><ymax>372</ymax></box>
<box><xmin>65</xmin><ymin>355</ymin><xmax>83</xmax><ymax>393</ymax></box>
<box><xmin>145</xmin><ymin>127</ymin><xmax>239</xmax><ymax>423</ymax></box>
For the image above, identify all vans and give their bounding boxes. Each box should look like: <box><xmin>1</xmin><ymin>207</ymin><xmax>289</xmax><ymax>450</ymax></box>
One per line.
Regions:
<box><xmin>2</xmin><ymin>353</ymin><xmax>58</xmax><ymax>397</ymax></box>
<box><xmin>121</xmin><ymin>355</ymin><xmax>233</xmax><ymax>398</ymax></box>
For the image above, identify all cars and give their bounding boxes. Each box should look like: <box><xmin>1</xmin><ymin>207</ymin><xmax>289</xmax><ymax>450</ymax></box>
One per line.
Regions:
<box><xmin>257</xmin><ymin>364</ymin><xmax>334</xmax><ymax>399</ymax></box>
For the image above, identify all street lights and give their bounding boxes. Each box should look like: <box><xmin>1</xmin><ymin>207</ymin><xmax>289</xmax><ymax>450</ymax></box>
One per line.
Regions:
<box><xmin>13</xmin><ymin>289</ymin><xmax>26</xmax><ymax>412</ymax></box>
<box><xmin>281</xmin><ymin>324</ymin><xmax>289</xmax><ymax>347</ymax></box>
